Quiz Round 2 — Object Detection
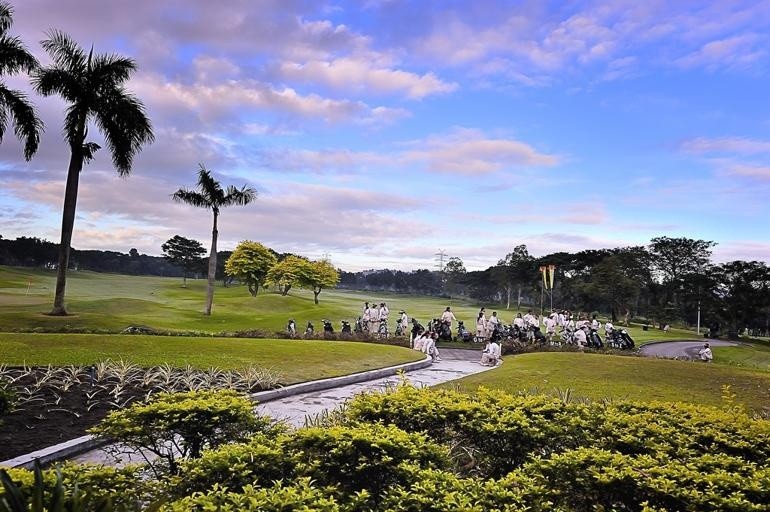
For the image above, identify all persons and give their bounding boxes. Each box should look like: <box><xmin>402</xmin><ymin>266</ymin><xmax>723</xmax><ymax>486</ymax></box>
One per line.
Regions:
<box><xmin>699</xmin><ymin>342</ymin><xmax>713</xmax><ymax>361</ymax></box>
<box><xmin>663</xmin><ymin>324</ymin><xmax>671</xmax><ymax>334</ymax></box>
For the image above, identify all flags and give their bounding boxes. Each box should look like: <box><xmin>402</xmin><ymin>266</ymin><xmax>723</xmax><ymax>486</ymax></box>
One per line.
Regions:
<box><xmin>550</xmin><ymin>271</ymin><xmax>554</xmax><ymax>288</ymax></box>
<box><xmin>543</xmin><ymin>271</ymin><xmax>548</xmax><ymax>289</ymax></box>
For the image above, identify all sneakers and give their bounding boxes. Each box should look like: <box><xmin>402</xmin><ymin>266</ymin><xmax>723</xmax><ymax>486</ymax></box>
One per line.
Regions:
<box><xmin>433</xmin><ymin>356</ymin><xmax>442</xmax><ymax>363</ymax></box>
<box><xmin>479</xmin><ymin>361</ymin><xmax>488</xmax><ymax>366</ymax></box>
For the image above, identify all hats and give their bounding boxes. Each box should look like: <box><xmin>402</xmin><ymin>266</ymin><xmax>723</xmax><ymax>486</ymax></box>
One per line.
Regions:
<box><xmin>398</xmin><ymin>309</ymin><xmax>405</xmax><ymax>314</ymax></box>
